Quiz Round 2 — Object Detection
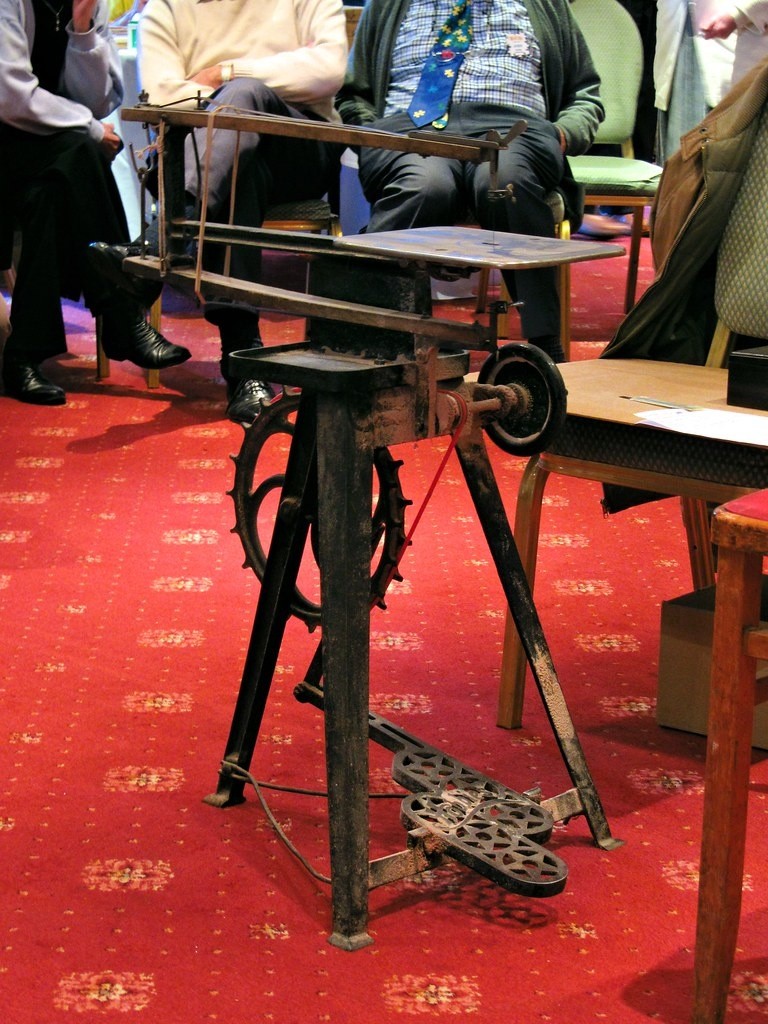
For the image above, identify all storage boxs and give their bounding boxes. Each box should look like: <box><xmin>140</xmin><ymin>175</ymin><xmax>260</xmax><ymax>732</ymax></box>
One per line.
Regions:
<box><xmin>657</xmin><ymin>574</ymin><xmax>768</xmax><ymax>754</ymax></box>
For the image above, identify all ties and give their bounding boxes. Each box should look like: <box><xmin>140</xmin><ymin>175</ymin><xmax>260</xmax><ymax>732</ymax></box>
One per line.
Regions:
<box><xmin>407</xmin><ymin>0</ymin><xmax>474</xmax><ymax>129</ymax></box>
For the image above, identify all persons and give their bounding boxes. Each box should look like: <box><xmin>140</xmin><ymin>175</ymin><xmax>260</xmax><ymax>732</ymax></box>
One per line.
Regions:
<box><xmin>1</xmin><ymin>0</ymin><xmax>350</xmax><ymax>423</ymax></box>
<box><xmin>336</xmin><ymin>0</ymin><xmax>768</xmax><ymax>368</ymax></box>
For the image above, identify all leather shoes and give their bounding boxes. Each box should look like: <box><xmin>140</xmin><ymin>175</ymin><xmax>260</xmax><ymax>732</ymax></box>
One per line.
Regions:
<box><xmin>224</xmin><ymin>376</ymin><xmax>273</xmax><ymax>422</ymax></box>
<box><xmin>91</xmin><ymin>239</ymin><xmax>162</xmax><ymax>307</ymax></box>
<box><xmin>3</xmin><ymin>359</ymin><xmax>65</xmax><ymax>401</ymax></box>
<box><xmin>102</xmin><ymin>316</ymin><xmax>191</xmax><ymax>369</ymax></box>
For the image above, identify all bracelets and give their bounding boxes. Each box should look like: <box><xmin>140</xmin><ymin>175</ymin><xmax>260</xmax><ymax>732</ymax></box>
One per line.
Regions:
<box><xmin>221</xmin><ymin>63</ymin><xmax>231</xmax><ymax>80</ymax></box>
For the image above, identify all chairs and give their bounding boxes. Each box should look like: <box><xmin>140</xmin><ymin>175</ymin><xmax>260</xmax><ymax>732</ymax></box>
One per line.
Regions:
<box><xmin>495</xmin><ymin>0</ymin><xmax>668</xmax><ymax>362</ymax></box>
<box><xmin>500</xmin><ymin>72</ymin><xmax>768</xmax><ymax>731</ymax></box>
<box><xmin>150</xmin><ymin>199</ymin><xmax>343</xmax><ymax>390</ymax></box>
